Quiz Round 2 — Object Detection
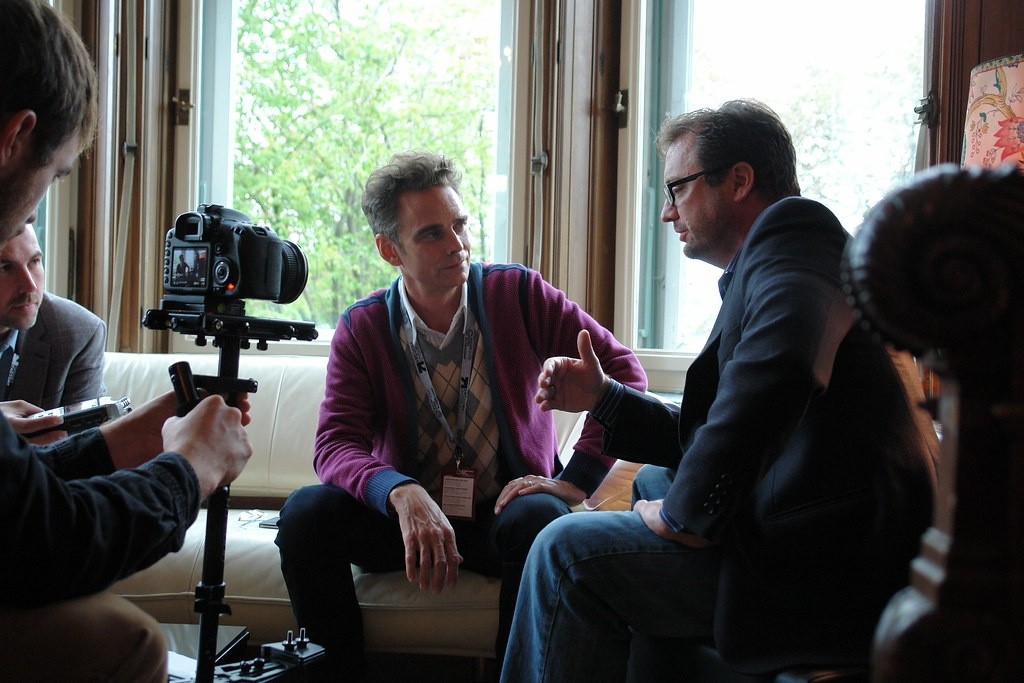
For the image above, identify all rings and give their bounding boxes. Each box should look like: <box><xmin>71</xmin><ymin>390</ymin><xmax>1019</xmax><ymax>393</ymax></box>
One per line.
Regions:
<box><xmin>528</xmin><ymin>480</ymin><xmax>534</xmax><ymax>486</ymax></box>
<box><xmin>551</xmin><ymin>385</ymin><xmax>556</xmax><ymax>399</ymax></box>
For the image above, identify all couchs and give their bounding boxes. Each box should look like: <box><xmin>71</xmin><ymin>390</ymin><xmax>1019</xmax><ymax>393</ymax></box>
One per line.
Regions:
<box><xmin>102</xmin><ymin>351</ymin><xmax>588</xmax><ymax>683</ymax></box>
<box><xmin>838</xmin><ymin>162</ymin><xmax>1024</xmax><ymax>683</ymax></box>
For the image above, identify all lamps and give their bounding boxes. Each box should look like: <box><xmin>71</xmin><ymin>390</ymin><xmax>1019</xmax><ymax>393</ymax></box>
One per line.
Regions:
<box><xmin>961</xmin><ymin>52</ymin><xmax>1024</xmax><ymax>171</ymax></box>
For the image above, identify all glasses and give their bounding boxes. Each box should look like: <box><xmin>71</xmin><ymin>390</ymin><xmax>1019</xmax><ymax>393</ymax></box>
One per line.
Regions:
<box><xmin>663</xmin><ymin>158</ymin><xmax>758</xmax><ymax>206</ymax></box>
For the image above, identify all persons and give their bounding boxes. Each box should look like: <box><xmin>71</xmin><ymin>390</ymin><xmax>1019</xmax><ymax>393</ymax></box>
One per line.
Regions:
<box><xmin>176</xmin><ymin>252</ymin><xmax>200</xmax><ymax>287</ymax></box>
<box><xmin>501</xmin><ymin>101</ymin><xmax>938</xmax><ymax>683</ymax></box>
<box><xmin>1</xmin><ymin>1</ymin><xmax>252</xmax><ymax>683</ymax></box>
<box><xmin>0</xmin><ymin>214</ymin><xmax>106</xmax><ymax>443</ymax></box>
<box><xmin>274</xmin><ymin>156</ymin><xmax>649</xmax><ymax>683</ymax></box>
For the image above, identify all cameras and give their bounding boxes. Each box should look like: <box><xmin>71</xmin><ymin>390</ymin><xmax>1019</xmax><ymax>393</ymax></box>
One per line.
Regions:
<box><xmin>164</xmin><ymin>204</ymin><xmax>309</xmax><ymax>305</ymax></box>
